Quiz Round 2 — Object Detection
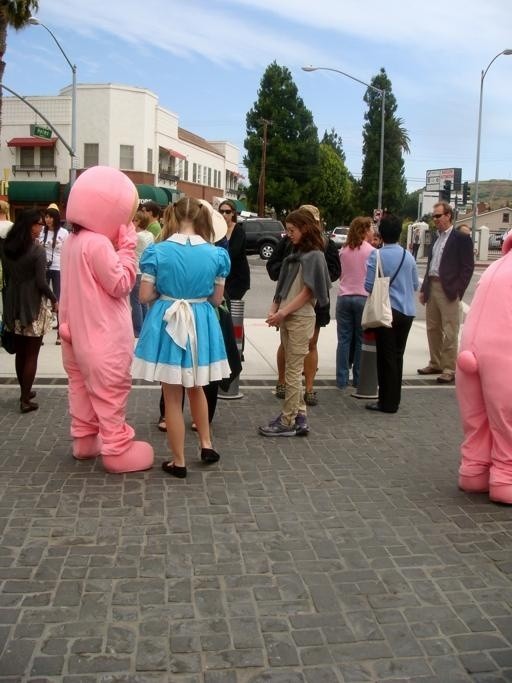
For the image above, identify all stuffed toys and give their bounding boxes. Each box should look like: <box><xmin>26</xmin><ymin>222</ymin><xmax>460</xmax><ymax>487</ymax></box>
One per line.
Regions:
<box><xmin>58</xmin><ymin>165</ymin><xmax>154</xmax><ymax>472</ymax></box>
<box><xmin>454</xmin><ymin>230</ymin><xmax>512</xmax><ymax>506</ymax></box>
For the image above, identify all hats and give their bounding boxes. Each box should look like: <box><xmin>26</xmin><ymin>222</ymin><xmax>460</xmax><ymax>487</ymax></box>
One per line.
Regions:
<box><xmin>46</xmin><ymin>203</ymin><xmax>59</xmax><ymax>212</ymax></box>
<box><xmin>198</xmin><ymin>199</ymin><xmax>228</xmax><ymax>243</ymax></box>
<box><xmin>299</xmin><ymin>205</ymin><xmax>320</xmax><ymax>221</ymax></box>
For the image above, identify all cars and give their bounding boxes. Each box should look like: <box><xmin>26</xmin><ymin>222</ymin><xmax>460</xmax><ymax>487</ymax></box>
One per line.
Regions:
<box><xmin>489</xmin><ymin>232</ymin><xmax>504</xmax><ymax>250</ymax></box>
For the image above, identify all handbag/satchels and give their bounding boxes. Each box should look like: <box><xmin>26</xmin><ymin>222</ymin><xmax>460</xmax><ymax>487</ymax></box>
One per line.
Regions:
<box><xmin>1</xmin><ymin>325</ymin><xmax>16</xmax><ymax>354</ymax></box>
<box><xmin>361</xmin><ymin>249</ymin><xmax>393</xmax><ymax>332</ymax></box>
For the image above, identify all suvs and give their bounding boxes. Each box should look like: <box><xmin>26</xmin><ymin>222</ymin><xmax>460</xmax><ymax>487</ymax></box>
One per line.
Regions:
<box><xmin>326</xmin><ymin>226</ymin><xmax>350</xmax><ymax>245</ymax></box>
<box><xmin>236</xmin><ymin>218</ymin><xmax>288</xmax><ymax>260</ymax></box>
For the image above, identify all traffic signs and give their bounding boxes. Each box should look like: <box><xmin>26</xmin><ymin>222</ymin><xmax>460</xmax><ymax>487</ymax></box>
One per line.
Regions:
<box><xmin>31</xmin><ymin>124</ymin><xmax>53</xmax><ymax>140</ymax></box>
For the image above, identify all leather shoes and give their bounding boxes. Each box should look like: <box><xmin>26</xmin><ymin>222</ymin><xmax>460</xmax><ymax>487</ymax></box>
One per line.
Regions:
<box><xmin>21</xmin><ymin>403</ymin><xmax>38</xmax><ymax>413</ymax></box>
<box><xmin>365</xmin><ymin>402</ymin><xmax>387</xmax><ymax>413</ymax></box>
<box><xmin>436</xmin><ymin>373</ymin><xmax>455</xmax><ymax>383</ymax></box>
<box><xmin>417</xmin><ymin>367</ymin><xmax>442</xmax><ymax>375</ymax></box>
<box><xmin>20</xmin><ymin>391</ymin><xmax>36</xmax><ymax>401</ymax></box>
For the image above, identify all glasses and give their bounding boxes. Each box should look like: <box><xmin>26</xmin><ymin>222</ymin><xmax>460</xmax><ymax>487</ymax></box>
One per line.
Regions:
<box><xmin>37</xmin><ymin>223</ymin><xmax>44</xmax><ymax>228</ymax></box>
<box><xmin>284</xmin><ymin>229</ymin><xmax>296</xmax><ymax>235</ymax></box>
<box><xmin>219</xmin><ymin>210</ymin><xmax>231</xmax><ymax>214</ymax></box>
<box><xmin>434</xmin><ymin>214</ymin><xmax>443</xmax><ymax>218</ymax></box>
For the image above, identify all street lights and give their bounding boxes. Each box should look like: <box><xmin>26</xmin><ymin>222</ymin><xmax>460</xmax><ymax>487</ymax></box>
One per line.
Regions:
<box><xmin>467</xmin><ymin>48</ymin><xmax>512</xmax><ymax>250</ymax></box>
<box><xmin>299</xmin><ymin>63</ymin><xmax>388</xmax><ymax>223</ymax></box>
<box><xmin>27</xmin><ymin>14</ymin><xmax>81</xmax><ymax>189</ymax></box>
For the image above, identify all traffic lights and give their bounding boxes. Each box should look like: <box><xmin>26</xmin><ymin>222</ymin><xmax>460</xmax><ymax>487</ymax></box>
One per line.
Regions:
<box><xmin>442</xmin><ymin>179</ymin><xmax>451</xmax><ymax>204</ymax></box>
<box><xmin>463</xmin><ymin>181</ymin><xmax>471</xmax><ymax>206</ymax></box>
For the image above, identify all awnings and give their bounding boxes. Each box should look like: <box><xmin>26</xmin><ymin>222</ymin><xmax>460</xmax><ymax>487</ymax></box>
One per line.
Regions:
<box><xmin>6</xmin><ymin>181</ymin><xmax>60</xmax><ymax>204</ymax></box>
<box><xmin>135</xmin><ymin>183</ymin><xmax>184</xmax><ymax>209</ymax></box>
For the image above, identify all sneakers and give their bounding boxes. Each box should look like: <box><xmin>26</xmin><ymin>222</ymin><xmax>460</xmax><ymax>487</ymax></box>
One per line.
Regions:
<box><xmin>269</xmin><ymin>415</ymin><xmax>310</xmax><ymax>437</ymax></box>
<box><xmin>162</xmin><ymin>461</ymin><xmax>186</xmax><ymax>477</ymax></box>
<box><xmin>258</xmin><ymin>421</ymin><xmax>297</xmax><ymax>437</ymax></box>
<box><xmin>201</xmin><ymin>448</ymin><xmax>220</xmax><ymax>463</ymax></box>
<box><xmin>51</xmin><ymin>310</ymin><xmax>58</xmax><ymax>329</ymax></box>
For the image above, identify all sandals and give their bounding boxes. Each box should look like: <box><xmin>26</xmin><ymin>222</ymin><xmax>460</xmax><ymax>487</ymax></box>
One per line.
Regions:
<box><xmin>304</xmin><ymin>391</ymin><xmax>317</xmax><ymax>405</ymax></box>
<box><xmin>276</xmin><ymin>384</ymin><xmax>286</xmax><ymax>398</ymax></box>
<box><xmin>191</xmin><ymin>419</ymin><xmax>196</xmax><ymax>431</ymax></box>
<box><xmin>158</xmin><ymin>416</ymin><xmax>167</xmax><ymax>432</ymax></box>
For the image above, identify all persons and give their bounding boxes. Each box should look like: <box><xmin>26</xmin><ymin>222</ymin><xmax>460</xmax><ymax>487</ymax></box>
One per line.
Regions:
<box><xmin>417</xmin><ymin>201</ymin><xmax>475</xmax><ymax>384</ymax></box>
<box><xmin>458</xmin><ymin>224</ymin><xmax>471</xmax><ymax>236</ymax></box>
<box><xmin>1</xmin><ymin>199</ymin><xmax>68</xmax><ymax>412</ymax></box>
<box><xmin>133</xmin><ymin>195</ymin><xmax>250</xmax><ymax>478</ymax></box>
<box><xmin>257</xmin><ymin>204</ymin><xmax>422</xmax><ymax>438</ymax></box>
